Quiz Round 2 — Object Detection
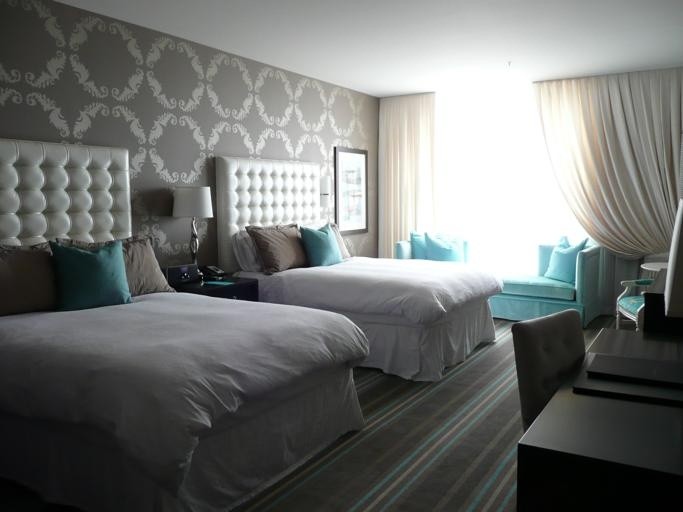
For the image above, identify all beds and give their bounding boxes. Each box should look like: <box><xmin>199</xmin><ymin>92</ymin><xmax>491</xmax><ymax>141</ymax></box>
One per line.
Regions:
<box><xmin>215</xmin><ymin>155</ymin><xmax>503</xmax><ymax>383</ymax></box>
<box><xmin>0</xmin><ymin>138</ymin><xmax>369</xmax><ymax>512</ymax></box>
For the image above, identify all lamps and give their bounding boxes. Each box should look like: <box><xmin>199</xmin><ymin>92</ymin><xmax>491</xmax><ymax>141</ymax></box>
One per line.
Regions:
<box><xmin>320</xmin><ymin>176</ymin><xmax>330</xmax><ymax>208</ymax></box>
<box><xmin>173</xmin><ymin>185</ymin><xmax>214</xmax><ymax>275</ymax></box>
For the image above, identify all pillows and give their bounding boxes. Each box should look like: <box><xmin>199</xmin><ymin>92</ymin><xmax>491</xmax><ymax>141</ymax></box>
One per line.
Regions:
<box><xmin>0</xmin><ymin>234</ymin><xmax>175</xmax><ymax>317</ymax></box>
<box><xmin>232</xmin><ymin>223</ymin><xmax>350</xmax><ymax>276</ymax></box>
<box><xmin>538</xmin><ymin>236</ymin><xmax>589</xmax><ymax>284</ymax></box>
<box><xmin>410</xmin><ymin>232</ymin><xmax>474</xmax><ymax>263</ymax></box>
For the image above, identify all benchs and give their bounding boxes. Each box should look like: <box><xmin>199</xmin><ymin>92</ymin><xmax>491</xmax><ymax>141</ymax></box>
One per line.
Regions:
<box><xmin>396</xmin><ymin>238</ymin><xmax>600</xmax><ymax>328</ymax></box>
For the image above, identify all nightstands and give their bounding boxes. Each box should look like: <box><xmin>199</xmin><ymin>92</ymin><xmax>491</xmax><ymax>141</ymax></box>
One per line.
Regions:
<box><xmin>171</xmin><ymin>274</ymin><xmax>259</xmax><ymax>303</ymax></box>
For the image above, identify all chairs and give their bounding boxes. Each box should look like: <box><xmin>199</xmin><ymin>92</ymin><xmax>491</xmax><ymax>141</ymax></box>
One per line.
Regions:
<box><xmin>511</xmin><ymin>309</ymin><xmax>585</xmax><ymax>435</ymax></box>
<box><xmin>615</xmin><ymin>269</ymin><xmax>668</xmax><ymax>332</ymax></box>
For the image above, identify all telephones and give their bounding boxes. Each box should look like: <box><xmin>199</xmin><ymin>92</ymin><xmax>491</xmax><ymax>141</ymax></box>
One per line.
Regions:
<box><xmin>198</xmin><ymin>265</ymin><xmax>225</xmax><ymax>277</ymax></box>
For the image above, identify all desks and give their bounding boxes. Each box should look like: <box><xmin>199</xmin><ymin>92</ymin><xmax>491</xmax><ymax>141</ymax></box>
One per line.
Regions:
<box><xmin>515</xmin><ymin>328</ymin><xmax>683</xmax><ymax>512</ymax></box>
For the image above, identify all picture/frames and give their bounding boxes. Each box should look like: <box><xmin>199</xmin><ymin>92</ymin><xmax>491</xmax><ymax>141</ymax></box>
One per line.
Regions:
<box><xmin>334</xmin><ymin>146</ymin><xmax>368</xmax><ymax>236</ymax></box>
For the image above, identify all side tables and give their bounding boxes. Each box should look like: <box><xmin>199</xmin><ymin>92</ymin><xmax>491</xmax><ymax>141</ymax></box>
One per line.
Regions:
<box><xmin>640</xmin><ymin>262</ymin><xmax>669</xmax><ymax>280</ymax></box>
